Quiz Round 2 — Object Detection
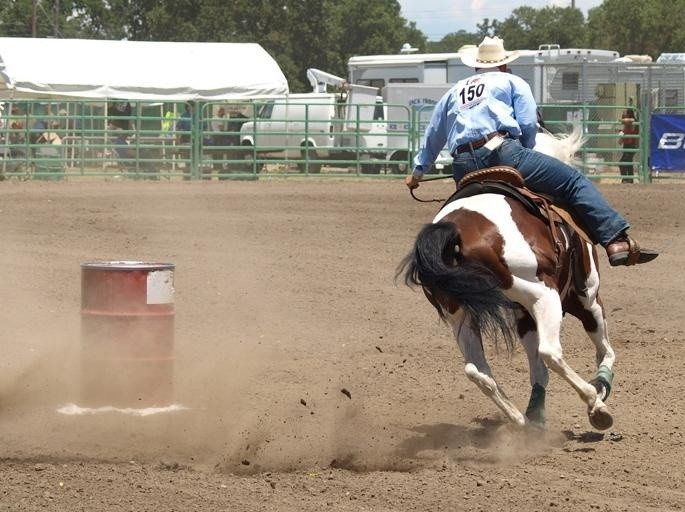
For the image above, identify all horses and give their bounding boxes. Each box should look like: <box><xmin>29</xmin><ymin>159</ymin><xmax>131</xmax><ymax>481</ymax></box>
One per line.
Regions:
<box><xmin>391</xmin><ymin>116</ymin><xmax>617</xmax><ymax>448</ymax></box>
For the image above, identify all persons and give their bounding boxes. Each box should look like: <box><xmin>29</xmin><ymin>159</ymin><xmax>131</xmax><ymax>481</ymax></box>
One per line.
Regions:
<box><xmin>617</xmin><ymin>106</ymin><xmax>640</xmax><ymax>183</ymax></box>
<box><xmin>404</xmin><ymin>36</ymin><xmax>659</xmax><ymax>267</ymax></box>
<box><xmin>1</xmin><ymin>99</ymin><xmax>252</xmax><ymax>182</ymax></box>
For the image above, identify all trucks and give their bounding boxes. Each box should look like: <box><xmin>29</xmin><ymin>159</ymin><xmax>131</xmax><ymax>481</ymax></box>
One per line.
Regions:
<box><xmin>241</xmin><ymin>68</ymin><xmax>457</xmax><ymax>173</ymax></box>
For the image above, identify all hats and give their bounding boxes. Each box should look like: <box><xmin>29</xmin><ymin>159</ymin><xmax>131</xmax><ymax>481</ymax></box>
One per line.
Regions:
<box><xmin>459</xmin><ymin>36</ymin><xmax>520</xmax><ymax>68</ymax></box>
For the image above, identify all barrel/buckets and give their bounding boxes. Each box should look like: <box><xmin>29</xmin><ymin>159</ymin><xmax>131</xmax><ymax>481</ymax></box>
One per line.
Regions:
<box><xmin>79</xmin><ymin>261</ymin><xmax>175</xmax><ymax>410</ymax></box>
<box><xmin>34</xmin><ymin>144</ymin><xmax>65</xmax><ymax>180</ymax></box>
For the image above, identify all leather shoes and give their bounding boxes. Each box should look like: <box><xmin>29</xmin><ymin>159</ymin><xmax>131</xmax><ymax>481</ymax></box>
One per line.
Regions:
<box><xmin>606</xmin><ymin>236</ymin><xmax>658</xmax><ymax>266</ymax></box>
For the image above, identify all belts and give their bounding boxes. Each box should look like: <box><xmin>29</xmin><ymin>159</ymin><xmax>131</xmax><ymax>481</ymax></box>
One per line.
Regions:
<box><xmin>455</xmin><ymin>130</ymin><xmax>507</xmax><ymax>154</ymax></box>
